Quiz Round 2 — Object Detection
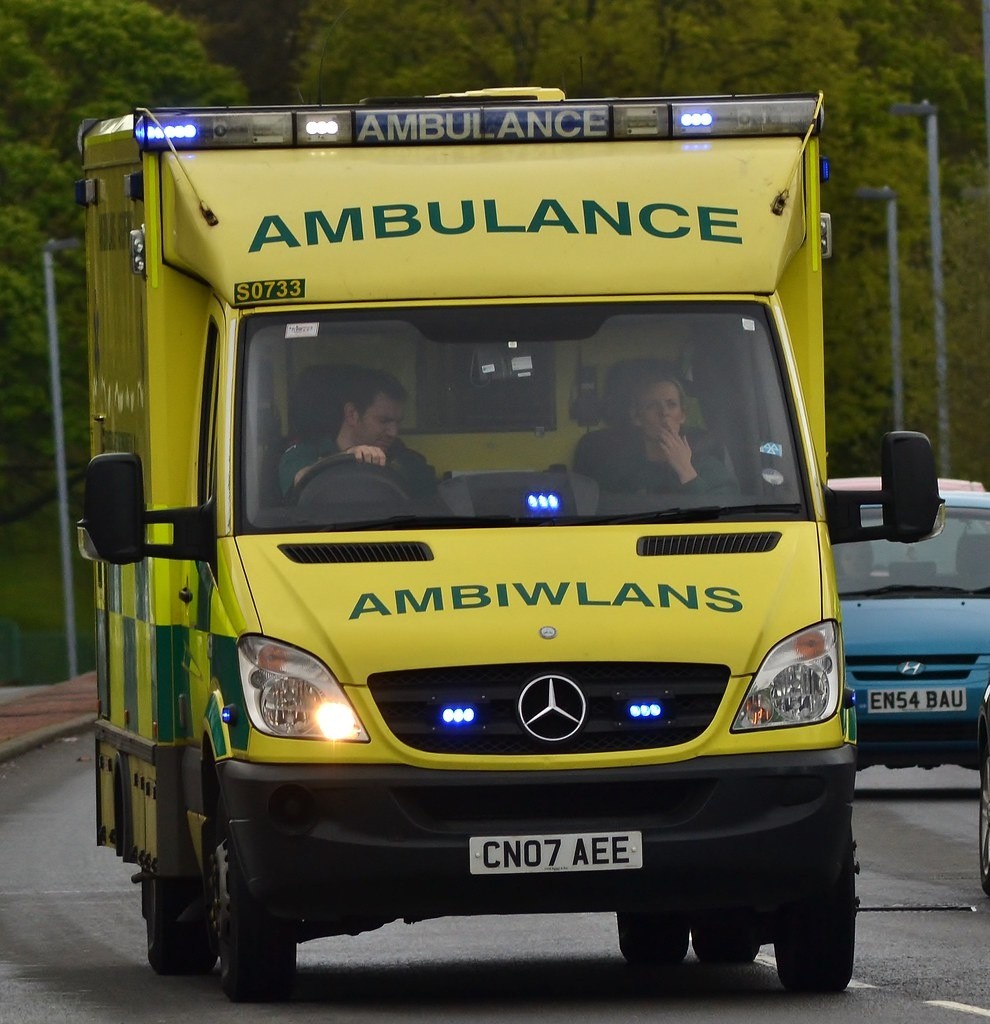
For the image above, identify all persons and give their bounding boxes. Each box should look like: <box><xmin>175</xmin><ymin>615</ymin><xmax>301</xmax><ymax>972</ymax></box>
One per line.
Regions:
<box><xmin>277</xmin><ymin>365</ymin><xmax>453</xmax><ymax>514</ymax></box>
<box><xmin>581</xmin><ymin>360</ymin><xmax>744</xmax><ymax>499</ymax></box>
<box><xmin>835</xmin><ymin>541</ymin><xmax>882</xmax><ymax>599</ymax></box>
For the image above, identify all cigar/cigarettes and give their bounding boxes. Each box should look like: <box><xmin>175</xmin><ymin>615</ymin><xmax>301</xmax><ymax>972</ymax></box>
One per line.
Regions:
<box><xmin>661</xmin><ymin>422</ymin><xmax>668</xmax><ymax>429</ymax></box>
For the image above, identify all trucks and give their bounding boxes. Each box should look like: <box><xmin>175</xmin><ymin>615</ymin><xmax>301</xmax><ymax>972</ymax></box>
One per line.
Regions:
<box><xmin>76</xmin><ymin>87</ymin><xmax>945</xmax><ymax>999</ymax></box>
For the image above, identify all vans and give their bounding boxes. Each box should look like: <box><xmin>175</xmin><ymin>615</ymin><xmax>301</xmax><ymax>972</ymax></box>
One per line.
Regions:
<box><xmin>826</xmin><ymin>478</ymin><xmax>990</xmax><ymax>767</ymax></box>
